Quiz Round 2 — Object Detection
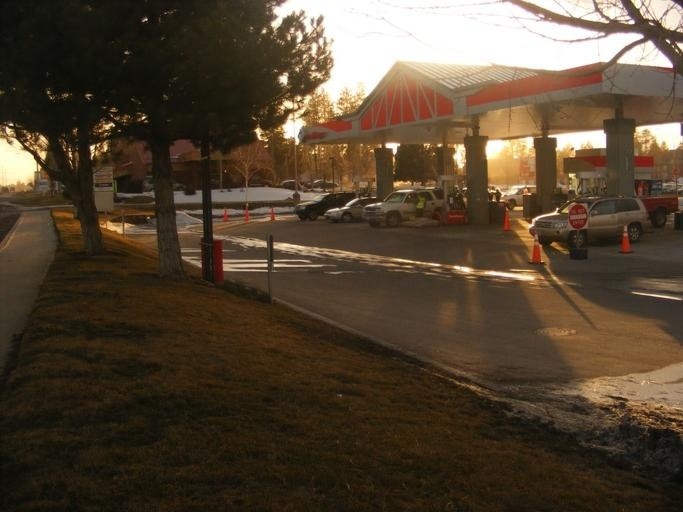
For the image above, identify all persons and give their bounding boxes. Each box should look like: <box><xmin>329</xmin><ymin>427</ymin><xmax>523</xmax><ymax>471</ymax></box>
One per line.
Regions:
<box><xmin>454</xmin><ymin>185</ymin><xmax>468</xmax><ymax>208</ymax></box>
<box><xmin>489</xmin><ymin>185</ymin><xmax>501</xmax><ymax>202</ymax></box>
<box><xmin>416</xmin><ymin>193</ymin><xmax>427</xmax><ymax>217</ymax></box>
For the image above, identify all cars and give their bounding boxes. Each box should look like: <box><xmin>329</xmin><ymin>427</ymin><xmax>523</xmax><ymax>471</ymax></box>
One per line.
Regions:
<box><xmin>248</xmin><ymin>177</ymin><xmax>340</xmax><ymax>191</ymax></box>
<box><xmin>294</xmin><ymin>184</ymin><xmax>502</xmax><ymax>228</ymax></box>
<box><xmin>662</xmin><ymin>183</ymin><xmax>683</xmax><ymax>194</ymax></box>
<box><xmin>528</xmin><ymin>193</ymin><xmax>653</xmax><ymax>246</ymax></box>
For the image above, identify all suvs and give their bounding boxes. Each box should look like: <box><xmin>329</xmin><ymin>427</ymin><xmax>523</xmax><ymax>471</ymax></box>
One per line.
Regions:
<box><xmin>502</xmin><ymin>185</ymin><xmax>537</xmax><ymax>209</ymax></box>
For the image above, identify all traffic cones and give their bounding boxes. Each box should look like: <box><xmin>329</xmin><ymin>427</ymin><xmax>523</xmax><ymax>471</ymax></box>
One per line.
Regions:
<box><xmin>502</xmin><ymin>210</ymin><xmax>512</xmax><ymax>231</ymax></box>
<box><xmin>619</xmin><ymin>224</ymin><xmax>635</xmax><ymax>254</ymax></box>
<box><xmin>528</xmin><ymin>232</ymin><xmax>545</xmax><ymax>265</ymax></box>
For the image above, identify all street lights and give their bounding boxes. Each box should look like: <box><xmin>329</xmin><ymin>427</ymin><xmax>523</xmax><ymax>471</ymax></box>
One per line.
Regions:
<box><xmin>329</xmin><ymin>157</ymin><xmax>335</xmax><ymax>192</ymax></box>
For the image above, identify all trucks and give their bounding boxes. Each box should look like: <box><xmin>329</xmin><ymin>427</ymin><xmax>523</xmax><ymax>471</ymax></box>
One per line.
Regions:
<box><xmin>639</xmin><ymin>195</ymin><xmax>679</xmax><ymax>228</ymax></box>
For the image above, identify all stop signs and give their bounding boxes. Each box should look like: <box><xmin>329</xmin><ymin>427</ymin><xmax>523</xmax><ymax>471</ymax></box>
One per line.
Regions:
<box><xmin>567</xmin><ymin>203</ymin><xmax>589</xmax><ymax>231</ymax></box>
